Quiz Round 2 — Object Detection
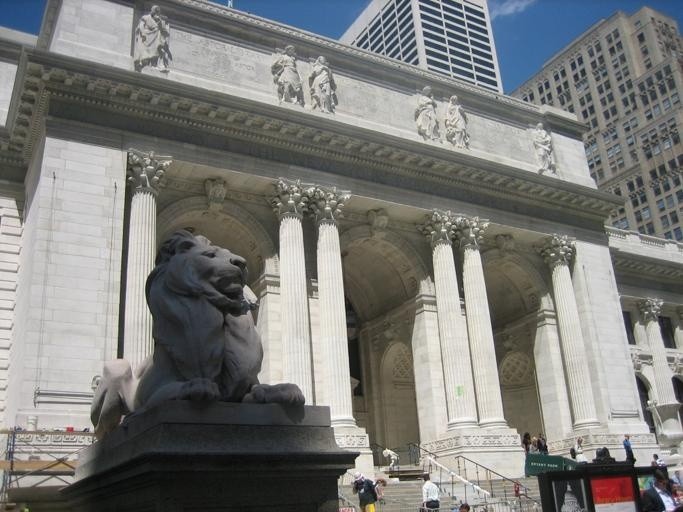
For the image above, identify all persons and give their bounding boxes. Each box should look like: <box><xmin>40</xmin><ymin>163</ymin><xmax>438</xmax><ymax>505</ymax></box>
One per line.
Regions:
<box><xmin>414</xmin><ymin>86</ymin><xmax>442</xmax><ymax>143</ymax></box>
<box><xmin>374</xmin><ymin>479</ymin><xmax>385</xmax><ymax>512</ymax></box>
<box><xmin>420</xmin><ymin>473</ymin><xmax>440</xmax><ymax>512</ymax></box>
<box><xmin>352</xmin><ymin>472</ymin><xmax>377</xmax><ymax>512</ymax></box>
<box><xmin>309</xmin><ymin>56</ymin><xmax>338</xmax><ymax>113</ymax></box>
<box><xmin>270</xmin><ymin>45</ymin><xmax>305</xmax><ymax>107</ymax></box>
<box><xmin>133</xmin><ymin>5</ymin><xmax>170</xmax><ymax>74</ymax></box>
<box><xmin>532</xmin><ymin>122</ymin><xmax>557</xmax><ymax>173</ymax></box>
<box><xmin>445</xmin><ymin>95</ymin><xmax>472</xmax><ymax>151</ymax></box>
<box><xmin>523</xmin><ymin>432</ymin><xmax>683</xmax><ymax>512</ymax></box>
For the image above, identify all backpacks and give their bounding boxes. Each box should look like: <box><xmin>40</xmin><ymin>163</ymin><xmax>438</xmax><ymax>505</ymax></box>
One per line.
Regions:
<box><xmin>569</xmin><ymin>447</ymin><xmax>576</xmax><ymax>459</ymax></box>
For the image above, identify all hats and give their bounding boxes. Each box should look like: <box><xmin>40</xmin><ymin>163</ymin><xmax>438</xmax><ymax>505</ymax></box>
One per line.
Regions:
<box><xmin>353</xmin><ymin>471</ymin><xmax>363</xmax><ymax>481</ymax></box>
